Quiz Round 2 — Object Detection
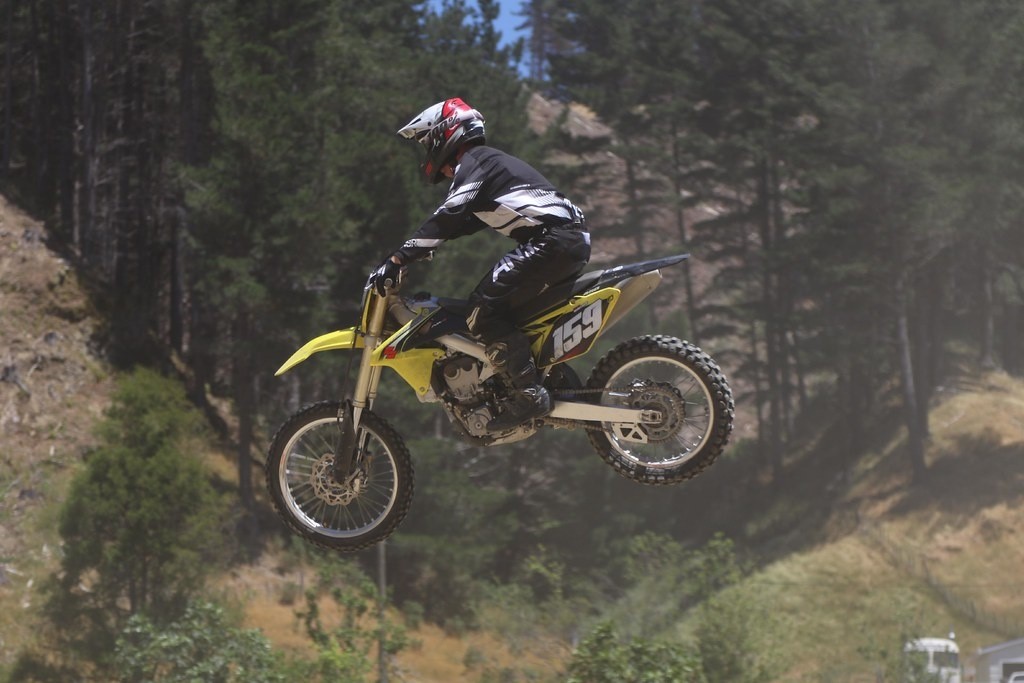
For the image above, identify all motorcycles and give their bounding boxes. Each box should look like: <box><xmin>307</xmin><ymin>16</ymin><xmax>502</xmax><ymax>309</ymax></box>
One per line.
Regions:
<box><xmin>264</xmin><ymin>252</ymin><xmax>738</xmax><ymax>553</ymax></box>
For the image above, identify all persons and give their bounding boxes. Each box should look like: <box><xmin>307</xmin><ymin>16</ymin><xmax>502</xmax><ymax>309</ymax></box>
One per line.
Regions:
<box><xmin>366</xmin><ymin>97</ymin><xmax>591</xmax><ymax>431</ymax></box>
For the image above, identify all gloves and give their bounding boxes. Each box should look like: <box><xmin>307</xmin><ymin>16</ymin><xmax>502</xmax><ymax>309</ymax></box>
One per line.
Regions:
<box><xmin>369</xmin><ymin>257</ymin><xmax>405</xmax><ymax>297</ymax></box>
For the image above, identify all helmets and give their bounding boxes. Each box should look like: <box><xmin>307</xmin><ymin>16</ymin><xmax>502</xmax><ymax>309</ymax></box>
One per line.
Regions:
<box><xmin>397</xmin><ymin>97</ymin><xmax>486</xmax><ymax>184</ymax></box>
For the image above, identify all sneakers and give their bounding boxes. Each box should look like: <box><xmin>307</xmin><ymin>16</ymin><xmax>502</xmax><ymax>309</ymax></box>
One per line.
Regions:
<box><xmin>486</xmin><ymin>383</ymin><xmax>555</xmax><ymax>430</ymax></box>
